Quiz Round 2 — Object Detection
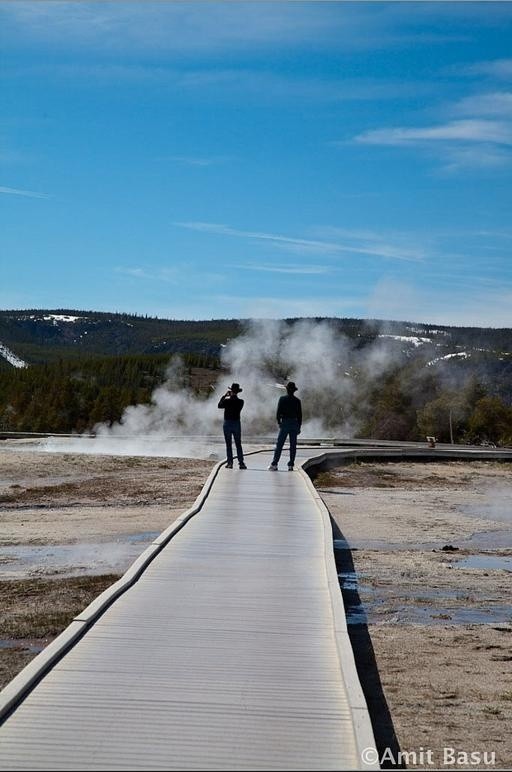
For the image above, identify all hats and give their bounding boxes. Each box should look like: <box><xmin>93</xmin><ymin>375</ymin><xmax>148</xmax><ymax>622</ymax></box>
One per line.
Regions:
<box><xmin>228</xmin><ymin>384</ymin><xmax>242</xmax><ymax>392</ymax></box>
<box><xmin>283</xmin><ymin>381</ymin><xmax>298</xmax><ymax>389</ymax></box>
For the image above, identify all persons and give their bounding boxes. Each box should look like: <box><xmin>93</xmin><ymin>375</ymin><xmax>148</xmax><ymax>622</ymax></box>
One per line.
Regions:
<box><xmin>218</xmin><ymin>384</ymin><xmax>247</xmax><ymax>469</ymax></box>
<box><xmin>267</xmin><ymin>381</ymin><xmax>302</xmax><ymax>471</ymax></box>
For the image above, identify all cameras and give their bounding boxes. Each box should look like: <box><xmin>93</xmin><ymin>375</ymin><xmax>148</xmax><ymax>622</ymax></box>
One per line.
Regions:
<box><xmin>228</xmin><ymin>391</ymin><xmax>232</xmax><ymax>395</ymax></box>
